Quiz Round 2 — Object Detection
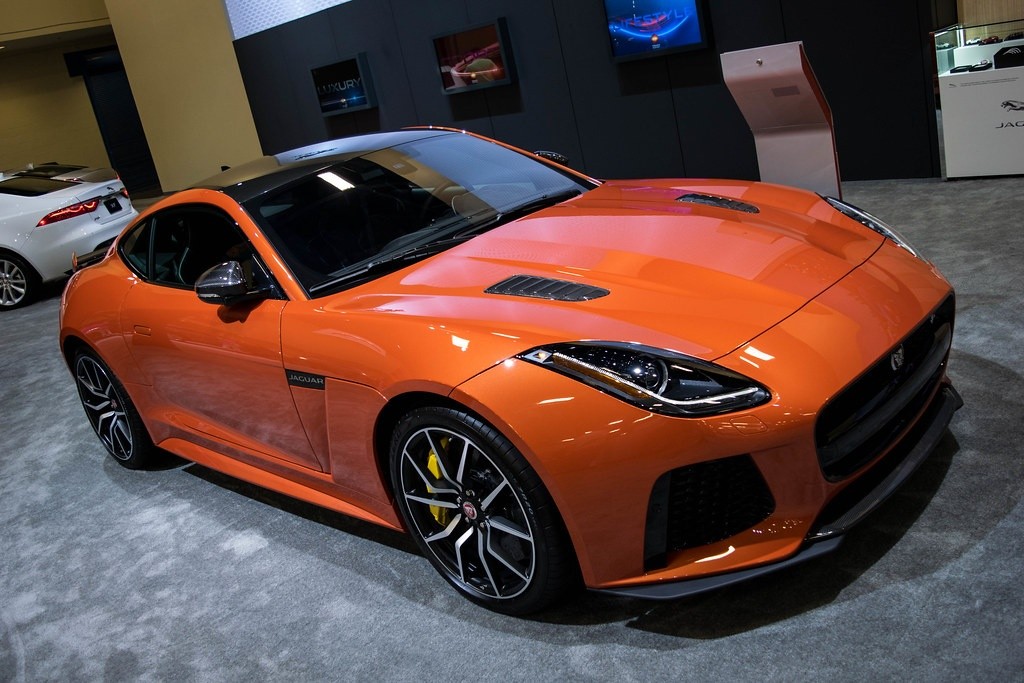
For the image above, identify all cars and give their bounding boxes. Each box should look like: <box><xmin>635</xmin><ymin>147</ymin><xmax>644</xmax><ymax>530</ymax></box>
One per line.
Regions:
<box><xmin>58</xmin><ymin>125</ymin><xmax>960</xmax><ymax>620</ymax></box>
<box><xmin>0</xmin><ymin>172</ymin><xmax>139</xmax><ymax>311</ymax></box>
<box><xmin>0</xmin><ymin>163</ymin><xmax>120</xmax><ymax>183</ymax></box>
<box><xmin>965</xmin><ymin>35</ymin><xmax>999</xmax><ymax>46</ymax></box>
